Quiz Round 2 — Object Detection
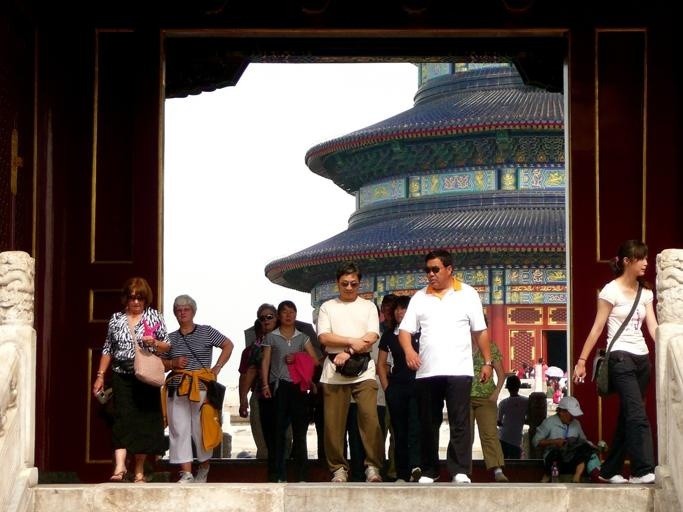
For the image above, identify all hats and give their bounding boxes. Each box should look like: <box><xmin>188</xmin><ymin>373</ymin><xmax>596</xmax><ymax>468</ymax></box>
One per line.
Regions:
<box><xmin>382</xmin><ymin>294</ymin><xmax>397</xmax><ymax>304</ymax></box>
<box><xmin>559</xmin><ymin>396</ymin><xmax>584</xmax><ymax>416</ymax></box>
<box><xmin>505</xmin><ymin>376</ymin><xmax>521</xmax><ymax>388</ymax></box>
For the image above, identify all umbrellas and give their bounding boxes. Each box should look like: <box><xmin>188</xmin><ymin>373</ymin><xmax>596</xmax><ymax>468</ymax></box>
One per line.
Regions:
<box><xmin>543</xmin><ymin>363</ymin><xmax>564</xmax><ymax>380</ymax></box>
<box><xmin>245</xmin><ymin>320</ymin><xmax>324</xmax><ymax>361</ymax></box>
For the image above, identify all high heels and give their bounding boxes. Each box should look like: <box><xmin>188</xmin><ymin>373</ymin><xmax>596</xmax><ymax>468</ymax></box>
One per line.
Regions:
<box><xmin>110</xmin><ymin>471</ymin><xmax>127</xmax><ymax>481</ymax></box>
<box><xmin>135</xmin><ymin>475</ymin><xmax>144</xmax><ymax>483</ymax></box>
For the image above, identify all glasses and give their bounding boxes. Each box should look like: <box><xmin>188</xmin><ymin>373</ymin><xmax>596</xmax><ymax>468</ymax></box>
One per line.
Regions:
<box><xmin>129</xmin><ymin>295</ymin><xmax>144</xmax><ymax>301</ymax></box>
<box><xmin>341</xmin><ymin>282</ymin><xmax>358</xmax><ymax>288</ymax></box>
<box><xmin>424</xmin><ymin>266</ymin><xmax>440</xmax><ymax>273</ymax></box>
<box><xmin>259</xmin><ymin>315</ymin><xmax>273</xmax><ymax>321</ymax></box>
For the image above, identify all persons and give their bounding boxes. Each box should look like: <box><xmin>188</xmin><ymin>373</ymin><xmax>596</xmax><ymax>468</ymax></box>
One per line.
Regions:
<box><xmin>315</xmin><ymin>341</ymin><xmax>348</xmax><ymax>459</ymax></box>
<box><xmin>471</xmin><ymin>312</ymin><xmax>508</xmax><ymax>481</ymax></box>
<box><xmin>238</xmin><ymin>319</ymin><xmax>294</xmax><ymax>460</ymax></box>
<box><xmin>240</xmin><ymin>303</ymin><xmax>280</xmax><ymax>481</ymax></box>
<box><xmin>317</xmin><ymin>261</ymin><xmax>383</xmax><ymax>482</ymax></box>
<box><xmin>513</xmin><ymin>358</ymin><xmax>568</xmax><ymax>404</ymax></box>
<box><xmin>159</xmin><ymin>295</ymin><xmax>235</xmax><ymax>483</ymax></box>
<box><xmin>93</xmin><ymin>278</ymin><xmax>172</xmax><ymax>485</ymax></box>
<box><xmin>573</xmin><ymin>239</ymin><xmax>659</xmax><ymax>484</ymax></box>
<box><xmin>497</xmin><ymin>375</ymin><xmax>530</xmax><ymax>459</ymax></box>
<box><xmin>261</xmin><ymin>300</ymin><xmax>320</xmax><ymax>483</ymax></box>
<box><xmin>398</xmin><ymin>249</ymin><xmax>493</xmax><ymax>485</ymax></box>
<box><xmin>376</xmin><ymin>296</ymin><xmax>421</xmax><ymax>482</ymax></box>
<box><xmin>376</xmin><ymin>294</ymin><xmax>398</xmax><ymax>482</ymax></box>
<box><xmin>347</xmin><ymin>303</ymin><xmax>392</xmax><ymax>483</ymax></box>
<box><xmin>532</xmin><ymin>398</ymin><xmax>598</xmax><ymax>483</ymax></box>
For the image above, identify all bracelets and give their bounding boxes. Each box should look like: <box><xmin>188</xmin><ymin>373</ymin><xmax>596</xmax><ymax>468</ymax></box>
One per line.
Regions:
<box><xmin>344</xmin><ymin>346</ymin><xmax>353</xmax><ymax>357</ymax></box>
<box><xmin>97</xmin><ymin>372</ymin><xmax>106</xmax><ymax>378</ymax></box>
<box><xmin>261</xmin><ymin>385</ymin><xmax>270</xmax><ymax>391</ymax></box>
<box><xmin>496</xmin><ymin>383</ymin><xmax>502</xmax><ymax>392</ymax></box>
<box><xmin>574</xmin><ymin>364</ymin><xmax>587</xmax><ymax>383</ymax></box>
<box><xmin>484</xmin><ymin>360</ymin><xmax>494</xmax><ymax>366</ymax></box>
<box><xmin>578</xmin><ymin>357</ymin><xmax>587</xmax><ymax>361</ymax></box>
<box><xmin>215</xmin><ymin>362</ymin><xmax>224</xmax><ymax>368</ymax></box>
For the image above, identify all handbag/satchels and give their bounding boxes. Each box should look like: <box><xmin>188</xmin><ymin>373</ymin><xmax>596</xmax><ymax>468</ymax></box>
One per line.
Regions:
<box><xmin>328</xmin><ymin>353</ymin><xmax>371</xmax><ymax>377</ymax></box>
<box><xmin>591</xmin><ymin>349</ymin><xmax>608</xmax><ymax>396</ymax></box>
<box><xmin>206</xmin><ymin>380</ymin><xmax>226</xmax><ymax>409</ymax></box>
<box><xmin>133</xmin><ymin>346</ymin><xmax>165</xmax><ymax>387</ymax></box>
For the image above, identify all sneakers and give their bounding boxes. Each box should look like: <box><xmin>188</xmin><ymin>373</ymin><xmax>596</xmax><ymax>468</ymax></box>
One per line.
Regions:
<box><xmin>452</xmin><ymin>473</ymin><xmax>471</xmax><ymax>483</ymax></box>
<box><xmin>365</xmin><ymin>466</ymin><xmax>383</xmax><ymax>482</ymax></box>
<box><xmin>195</xmin><ymin>464</ymin><xmax>210</xmax><ymax>483</ymax></box>
<box><xmin>412</xmin><ymin>466</ymin><xmax>422</xmax><ymax>482</ymax></box>
<box><xmin>494</xmin><ymin>472</ymin><xmax>508</xmax><ymax>482</ymax></box>
<box><xmin>331</xmin><ymin>467</ymin><xmax>348</xmax><ymax>482</ymax></box>
<box><xmin>176</xmin><ymin>470</ymin><xmax>194</xmax><ymax>483</ymax></box>
<box><xmin>598</xmin><ymin>474</ymin><xmax>628</xmax><ymax>484</ymax></box>
<box><xmin>418</xmin><ymin>475</ymin><xmax>440</xmax><ymax>484</ymax></box>
<box><xmin>628</xmin><ymin>473</ymin><xmax>655</xmax><ymax>483</ymax></box>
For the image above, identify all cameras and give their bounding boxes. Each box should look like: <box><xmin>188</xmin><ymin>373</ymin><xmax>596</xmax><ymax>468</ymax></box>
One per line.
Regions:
<box><xmin>96</xmin><ymin>388</ymin><xmax>113</xmax><ymax>403</ymax></box>
